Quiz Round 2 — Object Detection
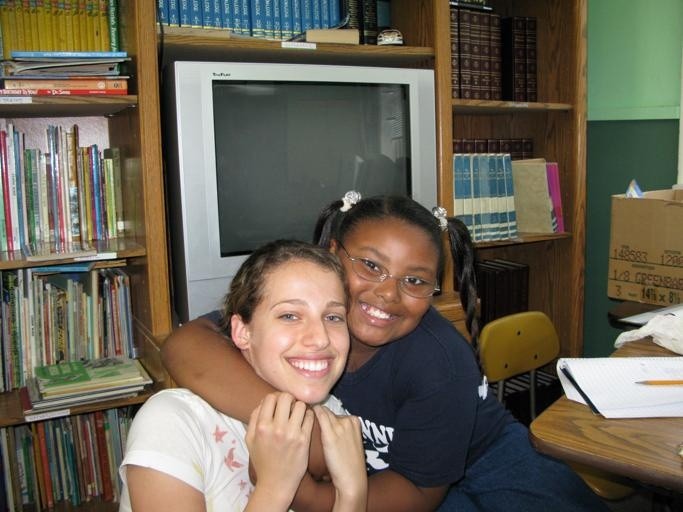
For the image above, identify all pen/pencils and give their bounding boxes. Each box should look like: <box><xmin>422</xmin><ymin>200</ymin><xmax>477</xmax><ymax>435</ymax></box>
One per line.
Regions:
<box><xmin>635</xmin><ymin>380</ymin><xmax>683</xmax><ymax>385</ymax></box>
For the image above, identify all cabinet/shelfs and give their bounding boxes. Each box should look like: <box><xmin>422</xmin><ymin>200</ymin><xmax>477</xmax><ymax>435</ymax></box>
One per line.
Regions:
<box><xmin>0</xmin><ymin>0</ymin><xmax>587</xmax><ymax>512</ymax></box>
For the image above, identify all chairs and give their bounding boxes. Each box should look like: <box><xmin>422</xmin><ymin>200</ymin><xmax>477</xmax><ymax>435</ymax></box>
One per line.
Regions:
<box><xmin>480</xmin><ymin>309</ymin><xmax>641</xmax><ymax>500</ymax></box>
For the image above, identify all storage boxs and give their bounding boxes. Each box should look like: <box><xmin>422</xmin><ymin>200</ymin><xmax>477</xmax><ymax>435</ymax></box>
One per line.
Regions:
<box><xmin>607</xmin><ymin>189</ymin><xmax>683</xmax><ymax>306</ymax></box>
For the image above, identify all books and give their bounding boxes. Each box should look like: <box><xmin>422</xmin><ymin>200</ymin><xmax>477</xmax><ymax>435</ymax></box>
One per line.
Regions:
<box><xmin>156</xmin><ymin>0</ymin><xmax>391</xmax><ymax>46</ymax></box>
<box><xmin>1</xmin><ymin>259</ymin><xmax>135</xmax><ymax>392</ymax></box>
<box><xmin>549</xmin><ymin>352</ymin><xmax>683</xmax><ymax>422</ymax></box>
<box><xmin>474</xmin><ymin>258</ymin><xmax>529</xmax><ymax>336</ymax></box>
<box><xmin>453</xmin><ymin>138</ymin><xmax>532</xmax><ymax>161</ymax></box>
<box><xmin>19</xmin><ymin>356</ymin><xmax>153</xmax><ymax>422</ymax></box>
<box><xmin>448</xmin><ymin>1</ymin><xmax>537</xmax><ymax>103</ymax></box>
<box><xmin>2</xmin><ymin>117</ymin><xmax>124</xmax><ymax>251</ymax></box>
<box><xmin>2</xmin><ymin>2</ymin><xmax>132</xmax><ymax>95</ymax></box>
<box><xmin>20</xmin><ymin>240</ymin><xmax>117</xmax><ymax>262</ymax></box>
<box><xmin>453</xmin><ymin>152</ymin><xmax>518</xmax><ymax>242</ymax></box>
<box><xmin>512</xmin><ymin>158</ymin><xmax>564</xmax><ymax>234</ymax></box>
<box><xmin>3</xmin><ymin>406</ymin><xmax>133</xmax><ymax>510</ymax></box>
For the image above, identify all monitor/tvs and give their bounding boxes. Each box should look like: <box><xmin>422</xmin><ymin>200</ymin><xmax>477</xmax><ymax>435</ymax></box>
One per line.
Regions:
<box><xmin>161</xmin><ymin>61</ymin><xmax>441</xmax><ymax>323</ymax></box>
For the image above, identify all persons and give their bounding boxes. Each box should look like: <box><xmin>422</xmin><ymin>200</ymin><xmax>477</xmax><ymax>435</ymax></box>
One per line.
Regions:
<box><xmin>114</xmin><ymin>238</ymin><xmax>369</xmax><ymax>512</ymax></box>
<box><xmin>158</xmin><ymin>188</ymin><xmax>612</xmax><ymax>511</ymax></box>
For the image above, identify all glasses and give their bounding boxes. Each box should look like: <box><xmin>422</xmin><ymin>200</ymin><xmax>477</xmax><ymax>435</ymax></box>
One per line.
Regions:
<box><xmin>333</xmin><ymin>239</ymin><xmax>443</xmax><ymax>303</ymax></box>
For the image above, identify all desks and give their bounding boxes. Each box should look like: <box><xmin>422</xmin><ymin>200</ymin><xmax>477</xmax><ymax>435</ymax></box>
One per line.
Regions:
<box><xmin>528</xmin><ymin>334</ymin><xmax>683</xmax><ymax>512</ymax></box>
<box><xmin>607</xmin><ymin>300</ymin><xmax>664</xmax><ymax>333</ymax></box>
